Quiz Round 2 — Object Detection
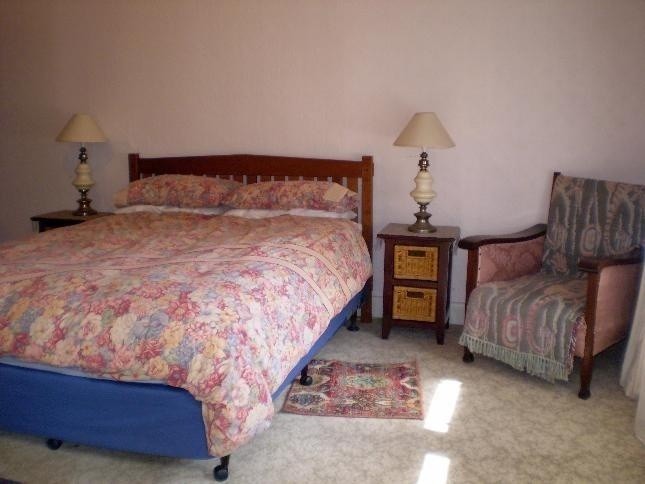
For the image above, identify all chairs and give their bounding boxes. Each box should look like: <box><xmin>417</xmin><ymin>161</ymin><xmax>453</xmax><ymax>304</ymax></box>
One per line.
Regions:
<box><xmin>458</xmin><ymin>171</ymin><xmax>645</xmax><ymax>400</ymax></box>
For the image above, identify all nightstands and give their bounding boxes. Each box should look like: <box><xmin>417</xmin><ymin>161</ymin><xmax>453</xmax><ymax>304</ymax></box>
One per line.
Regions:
<box><xmin>377</xmin><ymin>222</ymin><xmax>461</xmax><ymax>345</ymax></box>
<box><xmin>30</xmin><ymin>211</ymin><xmax>116</xmax><ymax>232</ymax></box>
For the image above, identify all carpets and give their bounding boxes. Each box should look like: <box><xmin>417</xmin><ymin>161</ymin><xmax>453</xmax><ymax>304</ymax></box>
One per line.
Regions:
<box><xmin>280</xmin><ymin>358</ymin><xmax>424</xmax><ymax>420</ymax></box>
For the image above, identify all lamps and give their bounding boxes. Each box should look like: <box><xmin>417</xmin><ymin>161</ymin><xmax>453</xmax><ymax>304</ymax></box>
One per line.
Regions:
<box><xmin>392</xmin><ymin>113</ymin><xmax>457</xmax><ymax>234</ymax></box>
<box><xmin>55</xmin><ymin>112</ymin><xmax>108</xmax><ymax>216</ymax></box>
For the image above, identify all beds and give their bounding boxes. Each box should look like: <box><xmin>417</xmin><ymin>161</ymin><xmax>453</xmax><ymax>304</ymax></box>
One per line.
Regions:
<box><xmin>1</xmin><ymin>151</ymin><xmax>375</xmax><ymax>481</ymax></box>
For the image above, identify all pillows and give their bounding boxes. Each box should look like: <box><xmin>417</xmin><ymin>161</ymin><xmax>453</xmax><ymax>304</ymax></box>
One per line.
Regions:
<box><xmin>112</xmin><ymin>173</ymin><xmax>240</xmax><ymax>208</ymax></box>
<box><xmin>542</xmin><ymin>173</ymin><xmax>645</xmax><ymax>272</ymax></box>
<box><xmin>223</xmin><ymin>180</ymin><xmax>358</xmax><ymax>213</ymax></box>
